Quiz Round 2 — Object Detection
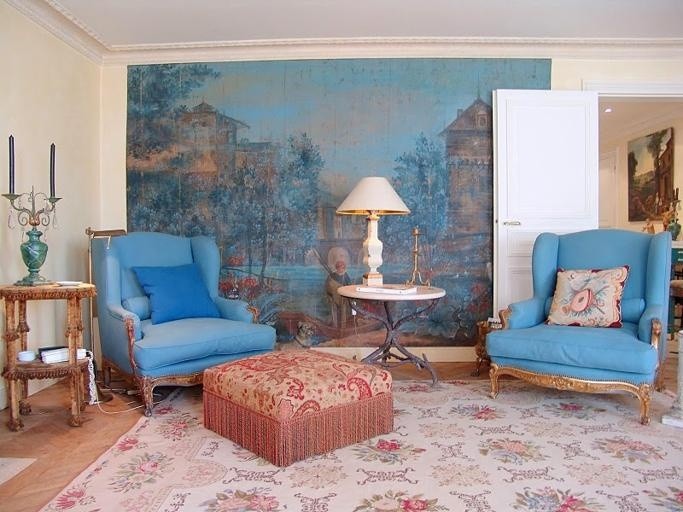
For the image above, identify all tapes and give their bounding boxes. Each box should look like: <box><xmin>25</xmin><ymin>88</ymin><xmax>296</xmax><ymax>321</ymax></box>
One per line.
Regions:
<box><xmin>18</xmin><ymin>351</ymin><xmax>35</xmax><ymax>362</ymax></box>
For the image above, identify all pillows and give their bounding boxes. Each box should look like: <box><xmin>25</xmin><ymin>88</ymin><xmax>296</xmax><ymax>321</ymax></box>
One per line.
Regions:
<box><xmin>123</xmin><ymin>296</ymin><xmax>152</xmax><ymax>321</ymax></box>
<box><xmin>544</xmin><ymin>265</ymin><xmax>631</xmax><ymax>327</ymax></box>
<box><xmin>543</xmin><ymin>297</ymin><xmax>645</xmax><ymax>324</ymax></box>
<box><xmin>130</xmin><ymin>261</ymin><xmax>220</xmax><ymax>325</ymax></box>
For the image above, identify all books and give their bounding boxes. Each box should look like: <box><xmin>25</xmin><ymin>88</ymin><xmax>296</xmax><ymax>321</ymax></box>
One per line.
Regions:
<box><xmin>356</xmin><ymin>283</ymin><xmax>418</xmax><ymax>295</ymax></box>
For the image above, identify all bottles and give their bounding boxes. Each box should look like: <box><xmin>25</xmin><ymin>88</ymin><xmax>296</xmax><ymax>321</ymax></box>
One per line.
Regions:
<box><xmin>667</xmin><ymin>218</ymin><xmax>682</xmax><ymax>240</ymax></box>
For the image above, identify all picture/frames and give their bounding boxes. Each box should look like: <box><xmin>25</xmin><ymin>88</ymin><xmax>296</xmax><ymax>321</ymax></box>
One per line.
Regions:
<box><xmin>627</xmin><ymin>127</ymin><xmax>673</xmax><ymax>222</ymax></box>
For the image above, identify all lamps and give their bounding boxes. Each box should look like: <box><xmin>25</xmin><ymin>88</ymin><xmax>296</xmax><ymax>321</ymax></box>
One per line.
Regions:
<box><xmin>335</xmin><ymin>177</ymin><xmax>411</xmax><ymax>286</ymax></box>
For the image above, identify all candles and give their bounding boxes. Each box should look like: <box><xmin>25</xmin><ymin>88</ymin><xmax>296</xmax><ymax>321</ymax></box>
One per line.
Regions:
<box><xmin>10</xmin><ymin>135</ymin><xmax>14</xmax><ymax>194</ymax></box>
<box><xmin>50</xmin><ymin>143</ymin><xmax>55</xmax><ymax>197</ymax></box>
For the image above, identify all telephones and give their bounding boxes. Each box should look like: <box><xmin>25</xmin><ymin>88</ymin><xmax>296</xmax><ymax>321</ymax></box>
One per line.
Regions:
<box><xmin>41</xmin><ymin>348</ymin><xmax>87</xmax><ymax>364</ymax></box>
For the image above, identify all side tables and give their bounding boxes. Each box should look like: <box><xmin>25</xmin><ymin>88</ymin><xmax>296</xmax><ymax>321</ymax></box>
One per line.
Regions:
<box><xmin>0</xmin><ymin>282</ymin><xmax>97</xmax><ymax>431</ymax></box>
<box><xmin>337</xmin><ymin>284</ymin><xmax>446</xmax><ymax>388</ymax></box>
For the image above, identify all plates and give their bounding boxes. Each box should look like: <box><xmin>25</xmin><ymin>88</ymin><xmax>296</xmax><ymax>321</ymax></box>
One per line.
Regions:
<box><xmin>57</xmin><ymin>281</ymin><xmax>82</xmax><ymax>286</ymax></box>
<box><xmin>18</xmin><ymin>351</ymin><xmax>35</xmax><ymax>361</ymax></box>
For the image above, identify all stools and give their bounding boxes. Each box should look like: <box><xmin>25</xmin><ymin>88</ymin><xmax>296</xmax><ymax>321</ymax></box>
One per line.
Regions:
<box><xmin>661</xmin><ymin>330</ymin><xmax>683</xmax><ymax>428</ymax></box>
<box><xmin>670</xmin><ymin>280</ymin><xmax>683</xmax><ymax>298</ymax></box>
<box><xmin>203</xmin><ymin>349</ymin><xmax>394</xmax><ymax>467</ymax></box>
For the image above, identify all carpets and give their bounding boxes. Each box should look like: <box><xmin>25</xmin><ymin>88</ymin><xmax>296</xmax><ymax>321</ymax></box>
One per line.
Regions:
<box><xmin>38</xmin><ymin>379</ymin><xmax>683</xmax><ymax>512</ymax></box>
<box><xmin>0</xmin><ymin>457</ymin><xmax>38</xmax><ymax>485</ymax></box>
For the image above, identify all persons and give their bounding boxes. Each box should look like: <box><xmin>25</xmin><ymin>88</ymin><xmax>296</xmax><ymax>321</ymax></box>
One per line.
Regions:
<box><xmin>324</xmin><ymin>260</ymin><xmax>355</xmax><ymax>300</ymax></box>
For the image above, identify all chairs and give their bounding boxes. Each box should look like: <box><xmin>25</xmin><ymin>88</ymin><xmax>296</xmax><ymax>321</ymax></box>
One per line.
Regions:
<box><xmin>91</xmin><ymin>232</ymin><xmax>276</xmax><ymax>417</ymax></box>
<box><xmin>485</xmin><ymin>228</ymin><xmax>672</xmax><ymax>424</ymax></box>
